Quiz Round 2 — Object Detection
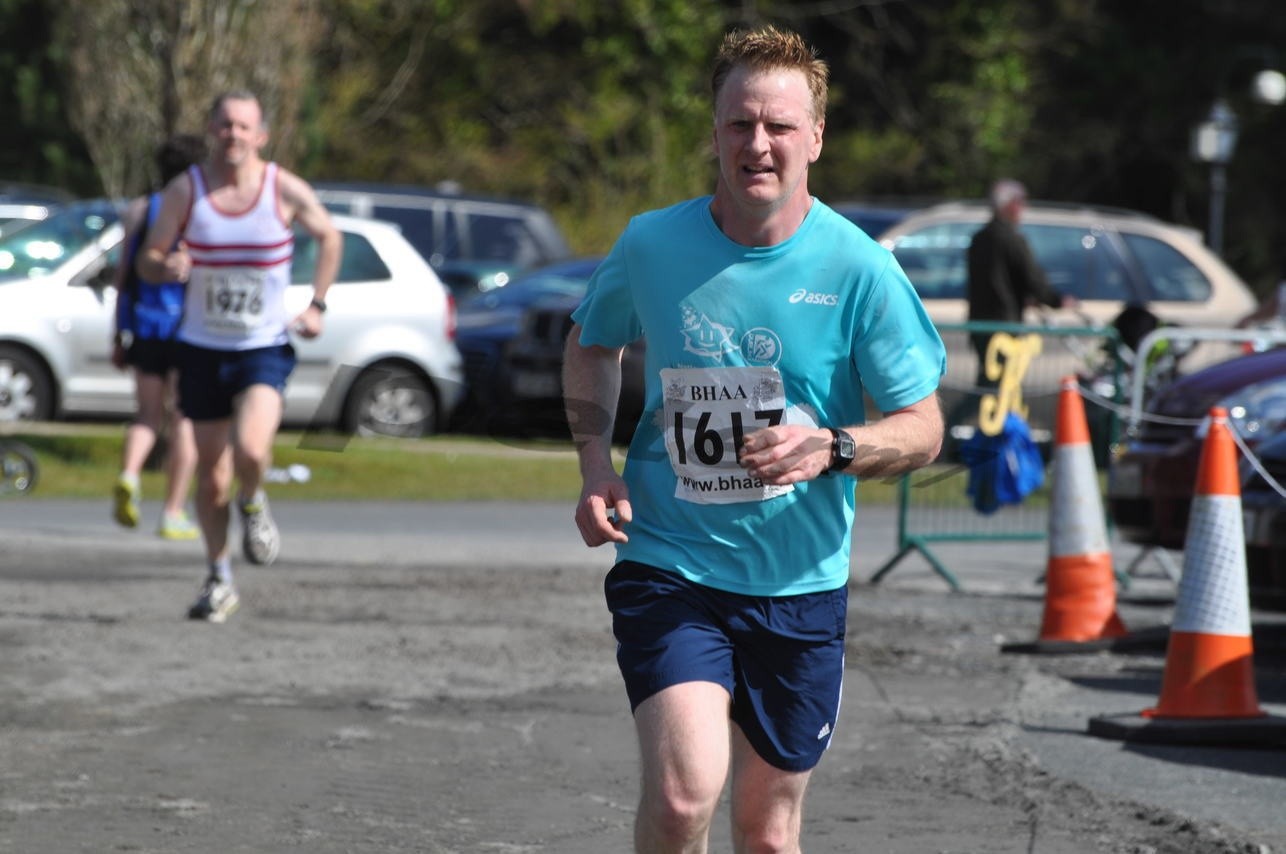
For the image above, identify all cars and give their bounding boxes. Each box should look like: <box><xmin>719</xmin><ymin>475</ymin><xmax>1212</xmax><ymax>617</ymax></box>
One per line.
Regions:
<box><xmin>1109</xmin><ymin>341</ymin><xmax>1286</xmax><ymax>550</ymax></box>
<box><xmin>0</xmin><ymin>193</ymin><xmax>67</xmax><ymax>241</ymax></box>
<box><xmin>458</xmin><ymin>201</ymin><xmax>915</xmax><ymax>428</ymax></box>
<box><xmin>875</xmin><ymin>203</ymin><xmax>1260</xmax><ymax>406</ymax></box>
<box><xmin>0</xmin><ymin>199</ymin><xmax>466</xmax><ymax>440</ymax></box>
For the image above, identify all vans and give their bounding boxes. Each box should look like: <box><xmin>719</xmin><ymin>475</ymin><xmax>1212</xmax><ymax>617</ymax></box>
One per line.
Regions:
<box><xmin>305</xmin><ymin>179</ymin><xmax>571</xmax><ymax>278</ymax></box>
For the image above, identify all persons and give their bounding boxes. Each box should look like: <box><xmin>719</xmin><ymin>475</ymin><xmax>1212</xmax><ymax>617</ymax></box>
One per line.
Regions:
<box><xmin>136</xmin><ymin>88</ymin><xmax>343</xmax><ymax>625</ymax></box>
<box><xmin>948</xmin><ymin>181</ymin><xmax>1070</xmax><ymax>432</ymax></box>
<box><xmin>112</xmin><ymin>133</ymin><xmax>205</xmax><ymax>540</ymax></box>
<box><xmin>561</xmin><ymin>22</ymin><xmax>948</xmax><ymax>854</ymax></box>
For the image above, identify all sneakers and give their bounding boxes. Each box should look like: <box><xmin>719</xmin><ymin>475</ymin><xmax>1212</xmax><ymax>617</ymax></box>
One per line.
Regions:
<box><xmin>237</xmin><ymin>490</ymin><xmax>282</xmax><ymax>568</ymax></box>
<box><xmin>160</xmin><ymin>510</ymin><xmax>201</xmax><ymax>541</ymax></box>
<box><xmin>114</xmin><ymin>473</ymin><xmax>139</xmax><ymax>529</ymax></box>
<box><xmin>189</xmin><ymin>577</ymin><xmax>242</xmax><ymax>623</ymax></box>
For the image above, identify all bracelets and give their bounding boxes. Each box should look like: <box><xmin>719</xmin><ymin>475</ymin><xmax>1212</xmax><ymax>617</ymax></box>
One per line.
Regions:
<box><xmin>312</xmin><ymin>296</ymin><xmax>326</xmax><ymax>313</ymax></box>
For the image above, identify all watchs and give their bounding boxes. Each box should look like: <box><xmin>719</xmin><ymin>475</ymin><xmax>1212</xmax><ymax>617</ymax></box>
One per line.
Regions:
<box><xmin>820</xmin><ymin>426</ymin><xmax>857</xmax><ymax>479</ymax></box>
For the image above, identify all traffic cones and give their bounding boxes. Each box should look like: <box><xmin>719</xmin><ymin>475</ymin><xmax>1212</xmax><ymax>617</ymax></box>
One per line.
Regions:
<box><xmin>1089</xmin><ymin>406</ymin><xmax>1286</xmax><ymax>748</ymax></box>
<box><xmin>999</xmin><ymin>375</ymin><xmax>1170</xmax><ymax>655</ymax></box>
